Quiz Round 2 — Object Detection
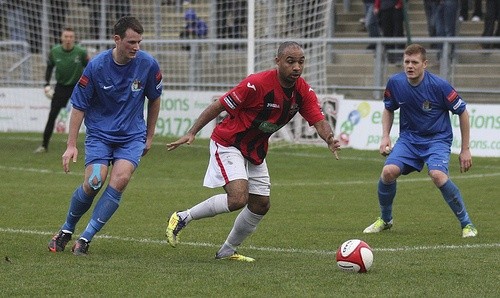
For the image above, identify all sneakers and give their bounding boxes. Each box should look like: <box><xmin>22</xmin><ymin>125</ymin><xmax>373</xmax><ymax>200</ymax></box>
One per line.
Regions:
<box><xmin>166</xmin><ymin>210</ymin><xmax>188</xmax><ymax>248</ymax></box>
<box><xmin>362</xmin><ymin>211</ymin><xmax>396</xmax><ymax>234</ymax></box>
<box><xmin>46</xmin><ymin>228</ymin><xmax>72</xmax><ymax>255</ymax></box>
<box><xmin>71</xmin><ymin>237</ymin><xmax>91</xmax><ymax>257</ymax></box>
<box><xmin>460</xmin><ymin>222</ymin><xmax>480</xmax><ymax>238</ymax></box>
<box><xmin>214</xmin><ymin>248</ymin><xmax>256</xmax><ymax>263</ymax></box>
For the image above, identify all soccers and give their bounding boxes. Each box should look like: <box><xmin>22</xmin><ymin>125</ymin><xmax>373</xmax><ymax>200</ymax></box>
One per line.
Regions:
<box><xmin>335</xmin><ymin>239</ymin><xmax>374</xmax><ymax>274</ymax></box>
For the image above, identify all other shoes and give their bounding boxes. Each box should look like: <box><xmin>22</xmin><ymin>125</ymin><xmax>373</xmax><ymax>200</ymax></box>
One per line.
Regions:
<box><xmin>458</xmin><ymin>13</ymin><xmax>483</xmax><ymax>23</ymax></box>
<box><xmin>32</xmin><ymin>143</ymin><xmax>49</xmax><ymax>156</ymax></box>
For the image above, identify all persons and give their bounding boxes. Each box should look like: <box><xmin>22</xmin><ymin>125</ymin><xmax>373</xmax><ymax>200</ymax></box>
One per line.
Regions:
<box><xmin>1</xmin><ymin>0</ymin><xmax>131</xmax><ymax>53</ymax></box>
<box><xmin>165</xmin><ymin>41</ymin><xmax>341</xmax><ymax>263</ymax></box>
<box><xmin>34</xmin><ymin>27</ymin><xmax>93</xmax><ymax>153</ymax></box>
<box><xmin>180</xmin><ymin>9</ymin><xmax>209</xmax><ymax>51</ymax></box>
<box><xmin>215</xmin><ymin>0</ymin><xmax>324</xmax><ymax>51</ymax></box>
<box><xmin>362</xmin><ymin>44</ymin><xmax>478</xmax><ymax>239</ymax></box>
<box><xmin>362</xmin><ymin>0</ymin><xmax>381</xmax><ymax>50</ymax></box>
<box><xmin>373</xmin><ymin>0</ymin><xmax>406</xmax><ymax>66</ymax></box>
<box><xmin>423</xmin><ymin>0</ymin><xmax>500</xmax><ymax>64</ymax></box>
<box><xmin>47</xmin><ymin>16</ymin><xmax>163</xmax><ymax>256</ymax></box>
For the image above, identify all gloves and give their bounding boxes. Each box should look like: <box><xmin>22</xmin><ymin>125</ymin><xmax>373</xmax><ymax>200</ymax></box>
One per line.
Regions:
<box><xmin>45</xmin><ymin>85</ymin><xmax>55</xmax><ymax>99</ymax></box>
